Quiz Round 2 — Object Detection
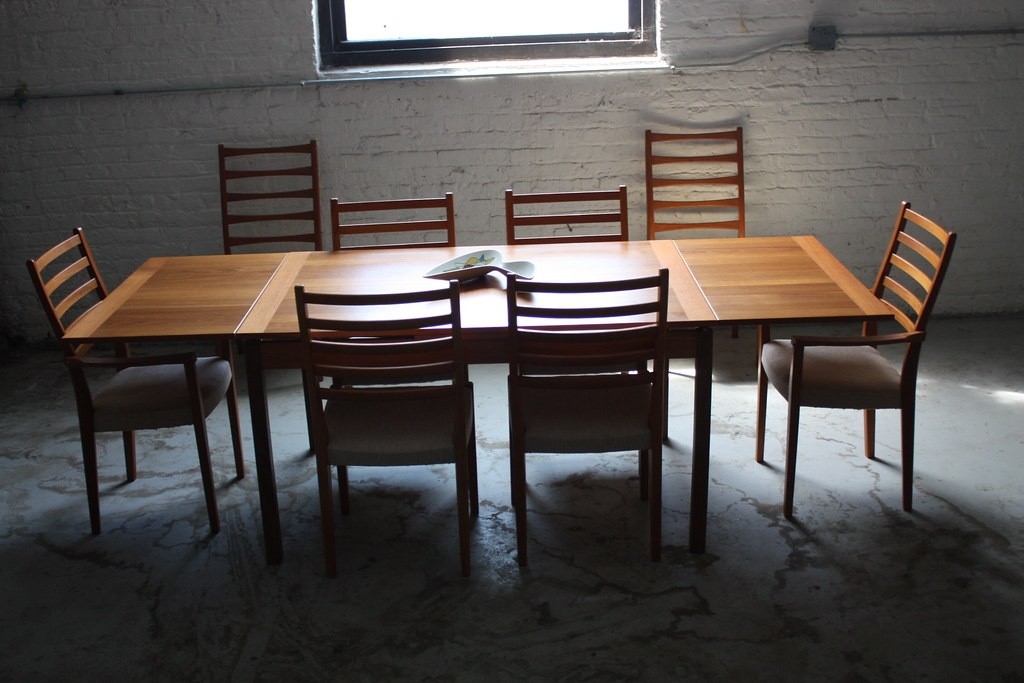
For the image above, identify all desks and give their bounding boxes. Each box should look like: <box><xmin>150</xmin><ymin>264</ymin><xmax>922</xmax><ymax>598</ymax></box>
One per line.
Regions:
<box><xmin>62</xmin><ymin>234</ymin><xmax>895</xmax><ymax>552</ymax></box>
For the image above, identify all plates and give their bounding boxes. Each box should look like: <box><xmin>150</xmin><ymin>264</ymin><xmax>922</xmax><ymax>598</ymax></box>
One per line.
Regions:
<box><xmin>422</xmin><ymin>248</ymin><xmax>536</xmax><ymax>281</ymax></box>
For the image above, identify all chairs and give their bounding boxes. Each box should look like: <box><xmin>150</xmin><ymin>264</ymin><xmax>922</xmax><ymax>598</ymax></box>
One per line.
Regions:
<box><xmin>293</xmin><ymin>285</ymin><xmax>478</xmax><ymax>578</ymax></box>
<box><xmin>507</xmin><ymin>182</ymin><xmax>668</xmax><ymax>441</ymax></box>
<box><xmin>758</xmin><ymin>198</ymin><xmax>957</xmax><ymax>522</ymax></box>
<box><xmin>26</xmin><ymin>226</ymin><xmax>246</xmax><ymax>540</ymax></box>
<box><xmin>300</xmin><ymin>193</ymin><xmax>469</xmax><ymax>454</ymax></box>
<box><xmin>507</xmin><ymin>269</ymin><xmax>672</xmax><ymax>570</ymax></box>
